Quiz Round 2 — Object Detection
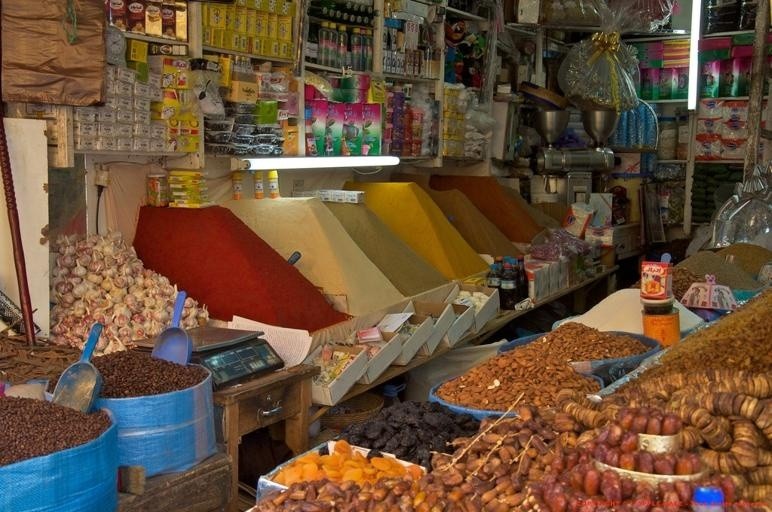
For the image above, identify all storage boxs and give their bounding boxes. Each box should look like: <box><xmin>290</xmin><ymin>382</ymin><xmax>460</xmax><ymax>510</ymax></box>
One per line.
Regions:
<box><xmin>440</xmin><ymin>303</ymin><xmax>475</xmax><ymax>348</ymax></box>
<box><xmin>445</xmin><ymin>283</ymin><xmax>501</xmax><ymax>336</ymax></box>
<box><xmin>311</xmin><ymin>341</ymin><xmax>370</xmax><ymax>408</ymax></box>
<box><xmin>378</xmin><ymin>314</ymin><xmax>435</xmax><ymax>367</ymax></box>
<box><xmin>348</xmin><ymin>331</ymin><xmax>403</xmax><ymax>384</ymax></box>
<box><xmin>402</xmin><ymin>298</ymin><xmax>455</xmax><ymax>359</ymax></box>
<box><xmin>527</xmin><ymin>247</ymin><xmax>571</xmax><ymax>304</ymax></box>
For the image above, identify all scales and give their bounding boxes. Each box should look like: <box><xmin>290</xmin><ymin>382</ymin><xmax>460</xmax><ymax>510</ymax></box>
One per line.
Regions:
<box><xmin>131</xmin><ymin>326</ymin><xmax>284</xmax><ymax>392</ymax></box>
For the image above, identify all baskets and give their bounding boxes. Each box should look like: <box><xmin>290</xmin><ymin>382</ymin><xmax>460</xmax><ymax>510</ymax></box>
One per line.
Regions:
<box><xmin>320</xmin><ymin>391</ymin><xmax>386</xmax><ymax>431</ymax></box>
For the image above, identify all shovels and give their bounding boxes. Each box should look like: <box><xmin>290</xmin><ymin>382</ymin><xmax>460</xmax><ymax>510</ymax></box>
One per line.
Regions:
<box><xmin>151</xmin><ymin>291</ymin><xmax>192</xmax><ymax>367</ymax></box>
<box><xmin>50</xmin><ymin>323</ymin><xmax>103</xmax><ymax>414</ymax></box>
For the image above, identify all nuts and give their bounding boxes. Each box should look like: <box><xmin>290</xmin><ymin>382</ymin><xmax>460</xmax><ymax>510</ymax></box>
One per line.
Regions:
<box><xmin>269</xmin><ymin>290</ymin><xmax>772</xmax><ymax>486</ymax></box>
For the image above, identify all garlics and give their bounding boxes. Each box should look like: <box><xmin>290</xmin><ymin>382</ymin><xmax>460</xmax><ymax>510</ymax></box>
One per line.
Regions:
<box><xmin>50</xmin><ymin>225</ymin><xmax>209</xmax><ymax>355</ymax></box>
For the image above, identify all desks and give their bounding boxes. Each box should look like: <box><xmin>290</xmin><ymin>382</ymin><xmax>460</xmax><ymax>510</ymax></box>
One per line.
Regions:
<box><xmin>214</xmin><ymin>361</ymin><xmax>323</xmax><ymax>512</ymax></box>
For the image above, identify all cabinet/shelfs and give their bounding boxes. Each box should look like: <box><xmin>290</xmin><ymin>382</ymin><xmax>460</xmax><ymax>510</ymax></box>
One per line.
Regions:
<box><xmin>2</xmin><ymin>2</ymin><xmax>768</xmax><ymax>237</ymax></box>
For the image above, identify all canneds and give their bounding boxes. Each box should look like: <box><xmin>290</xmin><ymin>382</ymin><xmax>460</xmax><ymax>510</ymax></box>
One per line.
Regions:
<box><xmin>146</xmin><ymin>174</ymin><xmax>170</xmax><ymax>208</ymax></box>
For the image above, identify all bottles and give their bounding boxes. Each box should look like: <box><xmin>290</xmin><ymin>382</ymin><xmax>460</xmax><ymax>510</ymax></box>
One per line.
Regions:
<box><xmin>487</xmin><ymin>253</ymin><xmax>528</xmax><ymax>309</ymax></box>
<box><xmin>305</xmin><ymin>21</ymin><xmax>372</xmax><ymax>73</ymax></box>
<box><xmin>230</xmin><ymin>171</ymin><xmax>280</xmax><ymax>201</ymax></box>
